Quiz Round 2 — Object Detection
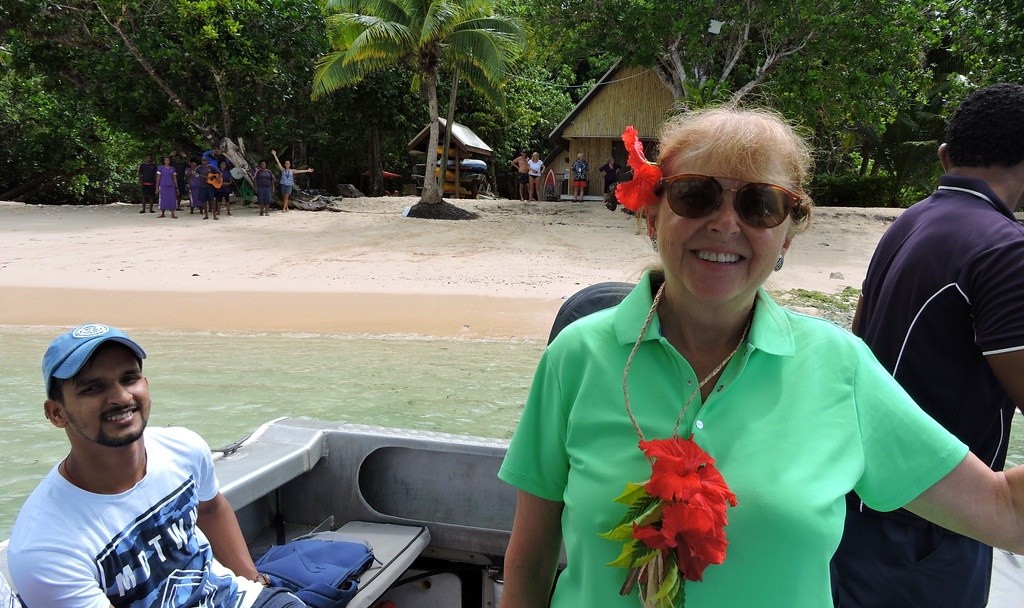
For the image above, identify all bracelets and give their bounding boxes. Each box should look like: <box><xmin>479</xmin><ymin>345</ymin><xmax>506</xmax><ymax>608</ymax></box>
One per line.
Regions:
<box><xmin>272</xmin><ymin>188</ymin><xmax>276</xmax><ymax>190</ymax></box>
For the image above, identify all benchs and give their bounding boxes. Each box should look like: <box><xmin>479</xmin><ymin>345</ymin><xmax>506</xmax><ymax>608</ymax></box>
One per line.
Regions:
<box><xmin>279</xmin><ymin>518</ymin><xmax>432</xmax><ymax>608</ymax></box>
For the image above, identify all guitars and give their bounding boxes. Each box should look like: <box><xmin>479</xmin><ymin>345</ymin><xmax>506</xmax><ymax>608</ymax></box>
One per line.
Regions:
<box><xmin>191</xmin><ymin>170</ymin><xmax>223</xmax><ymax>188</ymax></box>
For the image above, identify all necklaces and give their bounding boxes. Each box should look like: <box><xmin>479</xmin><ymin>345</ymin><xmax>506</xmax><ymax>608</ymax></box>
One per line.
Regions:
<box><xmin>621</xmin><ymin>276</ymin><xmax>753</xmax><ymax>469</ymax></box>
<box><xmin>285</xmin><ymin>168</ymin><xmax>291</xmax><ymax>178</ymax></box>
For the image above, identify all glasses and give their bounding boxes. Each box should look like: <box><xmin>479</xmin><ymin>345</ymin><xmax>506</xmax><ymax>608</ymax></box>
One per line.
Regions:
<box><xmin>201</xmin><ymin>160</ymin><xmax>207</xmax><ymax>161</ymax></box>
<box><xmin>654</xmin><ymin>172</ymin><xmax>803</xmax><ymax>229</ymax></box>
<box><xmin>522</xmin><ymin>151</ymin><xmax>526</xmax><ymax>154</ymax></box>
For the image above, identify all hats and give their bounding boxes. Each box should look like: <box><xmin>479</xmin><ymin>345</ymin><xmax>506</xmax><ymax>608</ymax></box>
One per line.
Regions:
<box><xmin>42</xmin><ymin>324</ymin><xmax>146</xmax><ymax>400</ymax></box>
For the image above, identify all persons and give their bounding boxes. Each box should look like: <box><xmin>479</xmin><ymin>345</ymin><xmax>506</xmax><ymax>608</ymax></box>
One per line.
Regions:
<box><xmin>635</xmin><ymin>206</ymin><xmax>651</xmax><ymax>237</ymax></box>
<box><xmin>137</xmin><ymin>147</ymin><xmax>233</xmax><ymax>220</ymax></box>
<box><xmin>6</xmin><ymin>322</ymin><xmax>315</xmax><ymax>608</ymax></box>
<box><xmin>511</xmin><ymin>148</ymin><xmax>537</xmax><ymax>201</ymax></box>
<box><xmin>492</xmin><ymin>104</ymin><xmax>1024</xmax><ymax>608</ymax></box>
<box><xmin>526</xmin><ymin>152</ymin><xmax>545</xmax><ymax>203</ymax></box>
<box><xmin>271</xmin><ymin>149</ymin><xmax>314</xmax><ymax>213</ymax></box>
<box><xmin>826</xmin><ymin>87</ymin><xmax>1024</xmax><ymax>608</ymax></box>
<box><xmin>570</xmin><ymin>151</ymin><xmax>590</xmax><ymax>202</ymax></box>
<box><xmin>254</xmin><ymin>160</ymin><xmax>276</xmax><ymax>217</ymax></box>
<box><xmin>597</xmin><ymin>157</ymin><xmax>621</xmax><ymax>201</ymax></box>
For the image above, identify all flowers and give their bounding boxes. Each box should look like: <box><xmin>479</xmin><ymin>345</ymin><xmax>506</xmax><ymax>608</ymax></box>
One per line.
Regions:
<box><xmin>596</xmin><ymin>434</ymin><xmax>737</xmax><ymax>608</ymax></box>
<box><xmin>615</xmin><ymin>124</ymin><xmax>662</xmax><ymax>211</ymax></box>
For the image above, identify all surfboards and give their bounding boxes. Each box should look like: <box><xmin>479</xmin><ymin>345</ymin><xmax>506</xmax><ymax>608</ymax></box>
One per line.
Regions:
<box><xmin>542</xmin><ymin>168</ymin><xmax>556</xmax><ymax>201</ymax></box>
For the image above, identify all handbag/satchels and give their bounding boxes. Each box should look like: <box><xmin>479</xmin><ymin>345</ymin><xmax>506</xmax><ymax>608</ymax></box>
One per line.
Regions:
<box><xmin>255</xmin><ymin>540</ymin><xmax>375</xmax><ymax>608</ymax></box>
